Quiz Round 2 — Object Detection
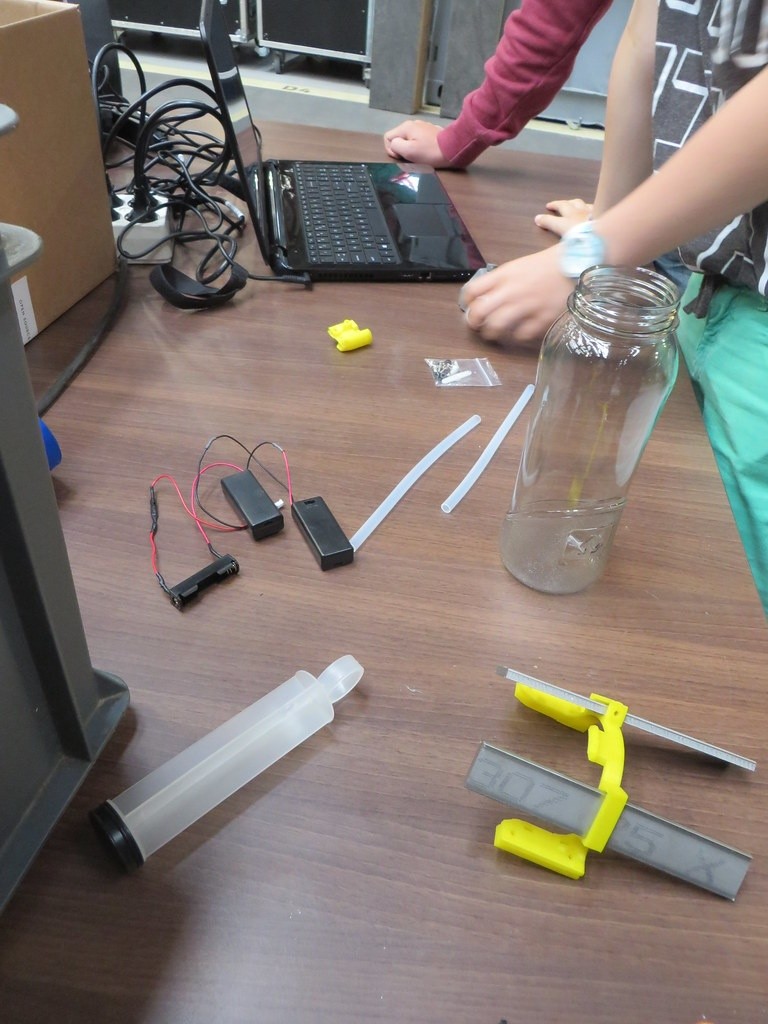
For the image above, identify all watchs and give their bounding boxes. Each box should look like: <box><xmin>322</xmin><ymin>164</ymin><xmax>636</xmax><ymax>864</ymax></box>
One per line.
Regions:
<box><xmin>557</xmin><ymin>217</ymin><xmax>610</xmax><ymax>292</ymax></box>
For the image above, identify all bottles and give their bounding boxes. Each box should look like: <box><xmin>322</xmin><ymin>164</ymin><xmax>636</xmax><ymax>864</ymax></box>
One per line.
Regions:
<box><xmin>497</xmin><ymin>265</ymin><xmax>684</xmax><ymax>595</ymax></box>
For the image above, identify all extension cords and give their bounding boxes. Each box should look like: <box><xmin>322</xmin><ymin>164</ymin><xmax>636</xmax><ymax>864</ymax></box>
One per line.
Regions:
<box><xmin>111</xmin><ymin>191</ymin><xmax>176</xmax><ymax>266</ymax></box>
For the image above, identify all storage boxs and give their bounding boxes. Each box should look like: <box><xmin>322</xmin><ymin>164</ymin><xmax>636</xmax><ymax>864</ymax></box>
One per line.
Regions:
<box><xmin>0</xmin><ymin>0</ymin><xmax>123</xmax><ymax>357</ymax></box>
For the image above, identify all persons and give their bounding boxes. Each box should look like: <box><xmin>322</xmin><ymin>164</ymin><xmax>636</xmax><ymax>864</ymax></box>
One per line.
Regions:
<box><xmin>373</xmin><ymin>0</ymin><xmax>617</xmax><ymax>175</ymax></box>
<box><xmin>461</xmin><ymin>0</ymin><xmax>767</xmax><ymax>624</ymax></box>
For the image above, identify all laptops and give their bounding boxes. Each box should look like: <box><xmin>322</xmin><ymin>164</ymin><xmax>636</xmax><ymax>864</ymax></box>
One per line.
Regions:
<box><xmin>198</xmin><ymin>0</ymin><xmax>489</xmax><ymax>279</ymax></box>
